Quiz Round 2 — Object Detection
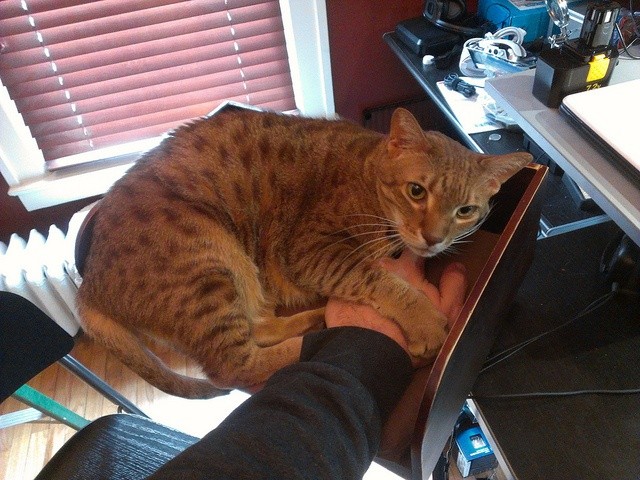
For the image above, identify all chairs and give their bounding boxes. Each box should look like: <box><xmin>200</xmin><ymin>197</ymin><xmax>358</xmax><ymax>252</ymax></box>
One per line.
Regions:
<box><xmin>0</xmin><ymin>290</ymin><xmax>204</xmax><ymax>480</ymax></box>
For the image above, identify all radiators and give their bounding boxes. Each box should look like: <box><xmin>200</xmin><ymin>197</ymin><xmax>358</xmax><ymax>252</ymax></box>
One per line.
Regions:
<box><xmin>0</xmin><ymin>215</ymin><xmax>89</xmax><ymax>336</ymax></box>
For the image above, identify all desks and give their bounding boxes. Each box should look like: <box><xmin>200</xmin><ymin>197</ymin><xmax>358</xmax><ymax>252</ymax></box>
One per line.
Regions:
<box><xmin>485</xmin><ymin>68</ymin><xmax>639</xmax><ymax>247</ymax></box>
<box><xmin>381</xmin><ymin>28</ymin><xmax>639</xmax><ymax>375</ymax></box>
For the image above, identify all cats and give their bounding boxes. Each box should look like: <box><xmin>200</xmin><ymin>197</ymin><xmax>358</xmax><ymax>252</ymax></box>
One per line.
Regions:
<box><xmin>72</xmin><ymin>104</ymin><xmax>537</xmax><ymax>401</ymax></box>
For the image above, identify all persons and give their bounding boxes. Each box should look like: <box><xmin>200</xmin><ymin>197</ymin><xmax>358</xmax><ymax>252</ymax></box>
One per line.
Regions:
<box><xmin>144</xmin><ymin>248</ymin><xmax>469</xmax><ymax>480</ymax></box>
